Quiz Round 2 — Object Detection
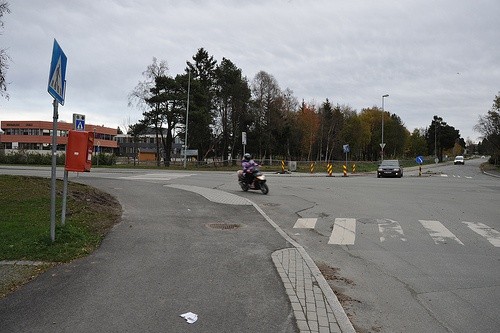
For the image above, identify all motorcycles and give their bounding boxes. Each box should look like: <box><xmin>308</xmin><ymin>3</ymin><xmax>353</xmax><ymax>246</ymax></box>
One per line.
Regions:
<box><xmin>236</xmin><ymin>165</ymin><xmax>269</xmax><ymax>194</ymax></box>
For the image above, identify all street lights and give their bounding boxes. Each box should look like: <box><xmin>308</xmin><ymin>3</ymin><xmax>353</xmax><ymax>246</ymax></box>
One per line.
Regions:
<box><xmin>381</xmin><ymin>94</ymin><xmax>390</xmax><ymax>162</ymax></box>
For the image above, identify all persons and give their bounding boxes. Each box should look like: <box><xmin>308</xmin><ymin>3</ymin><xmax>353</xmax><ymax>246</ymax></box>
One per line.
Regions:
<box><xmin>241</xmin><ymin>153</ymin><xmax>261</xmax><ymax>186</ymax></box>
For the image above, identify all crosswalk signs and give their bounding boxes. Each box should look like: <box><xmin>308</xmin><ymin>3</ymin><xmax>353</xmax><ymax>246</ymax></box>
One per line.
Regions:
<box><xmin>49</xmin><ymin>37</ymin><xmax>68</xmax><ymax>107</ymax></box>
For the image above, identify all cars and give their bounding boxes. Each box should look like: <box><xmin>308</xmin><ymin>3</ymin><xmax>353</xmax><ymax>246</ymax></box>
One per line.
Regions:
<box><xmin>454</xmin><ymin>156</ymin><xmax>465</xmax><ymax>165</ymax></box>
<box><xmin>377</xmin><ymin>160</ymin><xmax>404</xmax><ymax>178</ymax></box>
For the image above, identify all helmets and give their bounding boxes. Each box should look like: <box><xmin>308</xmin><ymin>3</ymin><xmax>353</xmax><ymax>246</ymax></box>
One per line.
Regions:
<box><xmin>244</xmin><ymin>153</ymin><xmax>251</xmax><ymax>161</ymax></box>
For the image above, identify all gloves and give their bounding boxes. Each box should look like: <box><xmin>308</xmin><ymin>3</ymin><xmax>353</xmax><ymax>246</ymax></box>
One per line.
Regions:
<box><xmin>255</xmin><ymin>164</ymin><xmax>258</xmax><ymax>167</ymax></box>
<box><xmin>247</xmin><ymin>170</ymin><xmax>250</xmax><ymax>172</ymax></box>
